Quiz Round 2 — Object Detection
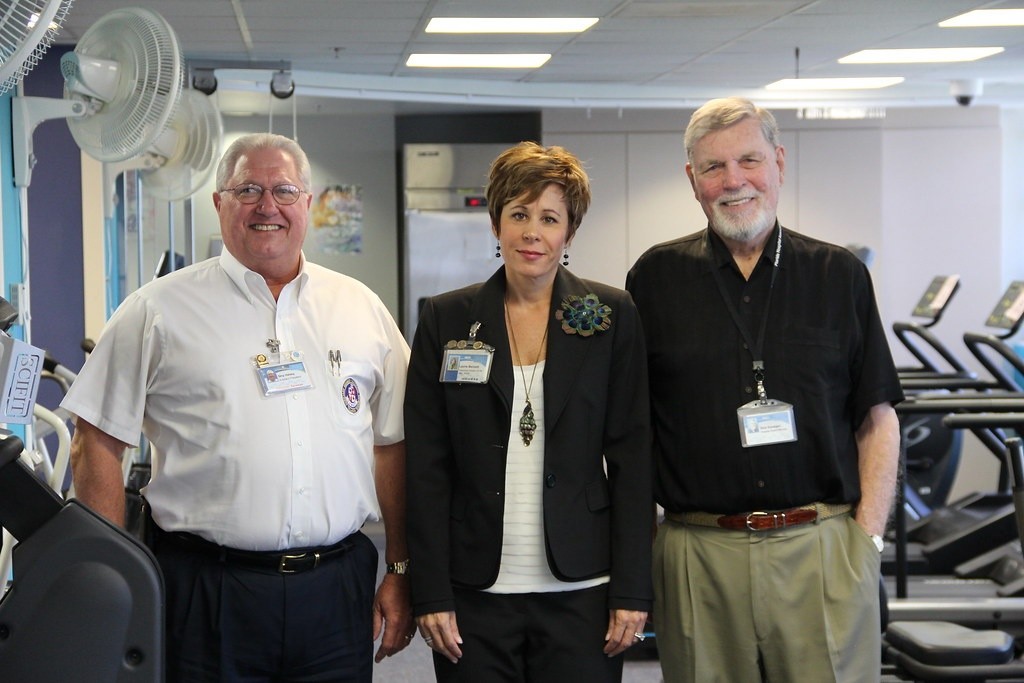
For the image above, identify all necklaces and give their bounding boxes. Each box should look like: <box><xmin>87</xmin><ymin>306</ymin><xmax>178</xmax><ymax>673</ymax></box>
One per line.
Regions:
<box><xmin>505</xmin><ymin>289</ymin><xmax>549</xmax><ymax>446</ymax></box>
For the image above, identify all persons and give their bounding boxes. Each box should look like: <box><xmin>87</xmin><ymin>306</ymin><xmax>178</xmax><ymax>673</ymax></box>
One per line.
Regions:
<box><xmin>624</xmin><ymin>99</ymin><xmax>903</xmax><ymax>683</ymax></box>
<box><xmin>403</xmin><ymin>142</ymin><xmax>652</xmax><ymax>681</ymax></box>
<box><xmin>62</xmin><ymin>134</ymin><xmax>416</xmax><ymax>683</ymax></box>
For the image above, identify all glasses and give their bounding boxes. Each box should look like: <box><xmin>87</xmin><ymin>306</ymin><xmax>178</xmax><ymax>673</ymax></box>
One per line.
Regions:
<box><xmin>218</xmin><ymin>183</ymin><xmax>307</xmax><ymax>206</ymax></box>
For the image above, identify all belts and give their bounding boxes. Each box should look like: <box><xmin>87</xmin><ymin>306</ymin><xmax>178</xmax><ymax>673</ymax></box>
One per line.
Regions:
<box><xmin>659</xmin><ymin>505</ymin><xmax>857</xmax><ymax>540</ymax></box>
<box><xmin>144</xmin><ymin>506</ymin><xmax>359</xmax><ymax>574</ymax></box>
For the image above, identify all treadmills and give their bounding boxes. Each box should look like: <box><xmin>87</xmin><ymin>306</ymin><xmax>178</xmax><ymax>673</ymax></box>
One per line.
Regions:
<box><xmin>877</xmin><ymin>315</ymin><xmax>1024</xmax><ymax>680</ymax></box>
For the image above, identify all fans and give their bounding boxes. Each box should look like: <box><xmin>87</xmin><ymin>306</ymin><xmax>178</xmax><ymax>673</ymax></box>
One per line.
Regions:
<box><xmin>0</xmin><ymin>0</ymin><xmax>74</xmax><ymax>97</ymax></box>
<box><xmin>10</xmin><ymin>6</ymin><xmax>186</xmax><ymax>188</ymax></box>
<box><xmin>102</xmin><ymin>88</ymin><xmax>223</xmax><ymax>219</ymax></box>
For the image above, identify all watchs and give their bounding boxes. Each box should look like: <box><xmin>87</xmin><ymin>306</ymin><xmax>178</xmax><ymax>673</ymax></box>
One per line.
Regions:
<box><xmin>870</xmin><ymin>535</ymin><xmax>885</xmax><ymax>553</ymax></box>
<box><xmin>385</xmin><ymin>559</ymin><xmax>410</xmax><ymax>577</ymax></box>
